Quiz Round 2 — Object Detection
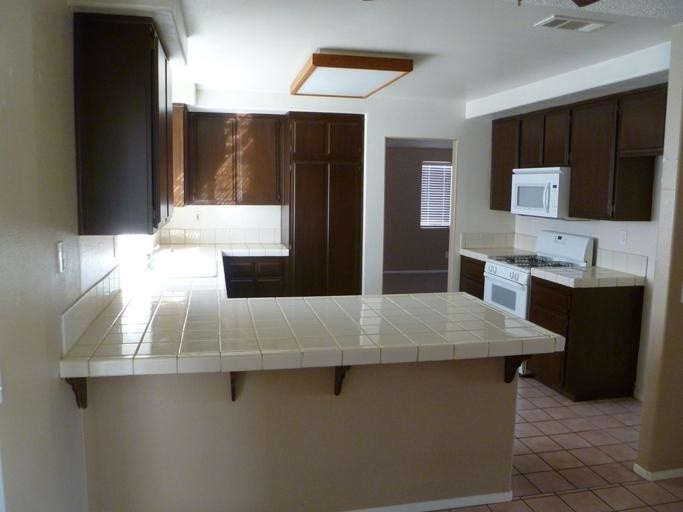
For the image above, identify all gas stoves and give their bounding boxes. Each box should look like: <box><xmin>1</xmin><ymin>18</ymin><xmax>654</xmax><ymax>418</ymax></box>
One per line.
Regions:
<box><xmin>484</xmin><ymin>252</ymin><xmax>585</xmax><ymax>286</ymax></box>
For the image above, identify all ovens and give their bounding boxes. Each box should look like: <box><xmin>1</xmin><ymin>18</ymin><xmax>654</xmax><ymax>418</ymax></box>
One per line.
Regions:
<box><xmin>483</xmin><ymin>271</ymin><xmax>527</xmax><ymax>375</ymax></box>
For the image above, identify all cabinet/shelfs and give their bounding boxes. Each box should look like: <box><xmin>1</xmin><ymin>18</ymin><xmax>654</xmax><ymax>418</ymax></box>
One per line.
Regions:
<box><xmin>188</xmin><ymin>112</ymin><xmax>280</xmax><ymax>206</ymax></box>
<box><xmin>489</xmin><ymin>115</ymin><xmax>520</xmax><ymax>213</ymax></box>
<box><xmin>224</xmin><ymin>256</ymin><xmax>290</xmax><ymax>298</ymax></box>
<box><xmin>458</xmin><ymin>255</ymin><xmax>485</xmax><ymax>301</ymax></box>
<box><xmin>566</xmin><ymin>85</ymin><xmax>653</xmax><ymax>221</ymax></box>
<box><xmin>526</xmin><ymin>277</ymin><xmax>645</xmax><ymax>401</ymax></box>
<box><xmin>519</xmin><ymin>104</ymin><xmax>570</xmax><ymax>167</ymax></box>
<box><xmin>280</xmin><ymin>111</ymin><xmax>365</xmax><ymax>163</ymax></box>
<box><xmin>72</xmin><ymin>10</ymin><xmax>176</xmax><ymax>235</ymax></box>
<box><xmin>615</xmin><ymin>81</ymin><xmax>668</xmax><ymax>159</ymax></box>
<box><xmin>280</xmin><ymin>160</ymin><xmax>364</xmax><ymax>296</ymax></box>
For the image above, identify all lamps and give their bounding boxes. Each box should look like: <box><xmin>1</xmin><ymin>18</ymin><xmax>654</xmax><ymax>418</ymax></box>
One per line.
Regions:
<box><xmin>290</xmin><ymin>49</ymin><xmax>414</xmax><ymax>99</ymax></box>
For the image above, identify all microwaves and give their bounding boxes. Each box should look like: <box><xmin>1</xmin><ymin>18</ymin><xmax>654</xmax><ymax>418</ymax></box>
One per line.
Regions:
<box><xmin>511</xmin><ymin>167</ymin><xmax>571</xmax><ymax>218</ymax></box>
